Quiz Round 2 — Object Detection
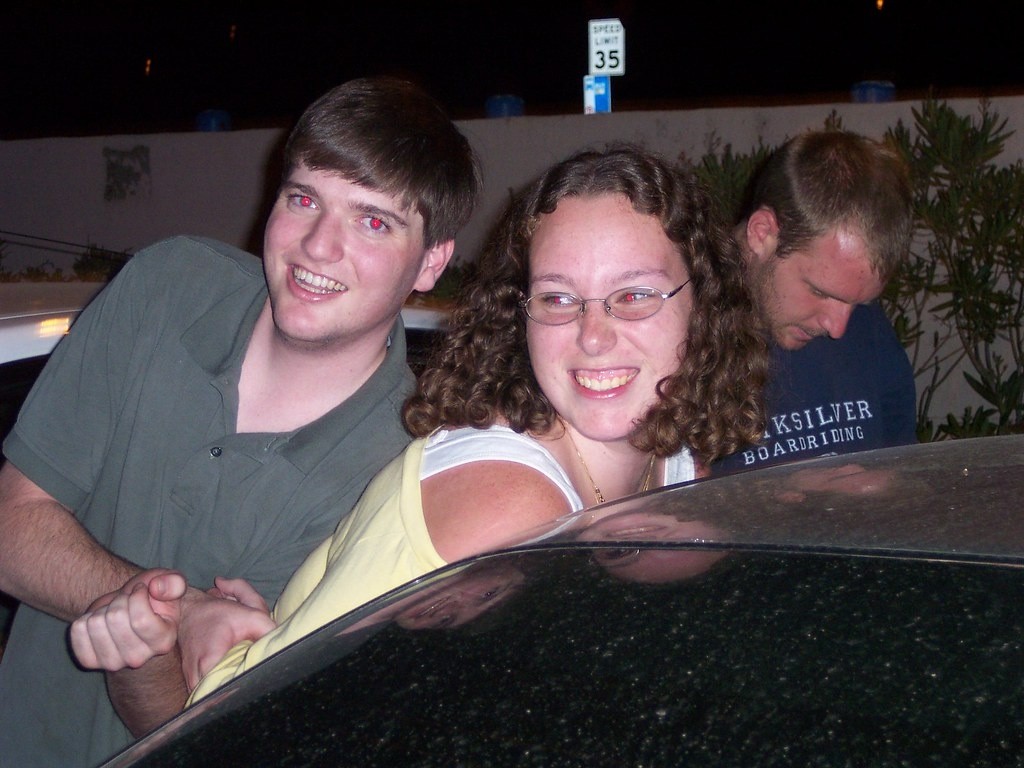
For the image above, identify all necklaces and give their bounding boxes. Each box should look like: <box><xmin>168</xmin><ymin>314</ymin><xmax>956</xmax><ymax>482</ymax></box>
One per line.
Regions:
<box><xmin>564</xmin><ymin>428</ymin><xmax>656</xmax><ymax>505</ymax></box>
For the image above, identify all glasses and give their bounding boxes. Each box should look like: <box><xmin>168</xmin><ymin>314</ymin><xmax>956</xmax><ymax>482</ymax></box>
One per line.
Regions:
<box><xmin>586</xmin><ymin>547</ymin><xmax>640</xmax><ymax>567</ymax></box>
<box><xmin>520</xmin><ymin>278</ymin><xmax>691</xmax><ymax>326</ymax></box>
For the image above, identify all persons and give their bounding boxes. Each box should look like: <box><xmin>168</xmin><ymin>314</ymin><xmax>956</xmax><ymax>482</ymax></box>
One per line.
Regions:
<box><xmin>713</xmin><ymin>129</ymin><xmax>918</xmax><ymax>479</ymax></box>
<box><xmin>183</xmin><ymin>141</ymin><xmax>774</xmax><ymax>714</ymax></box>
<box><xmin>0</xmin><ymin>75</ymin><xmax>486</xmax><ymax>767</ymax></box>
